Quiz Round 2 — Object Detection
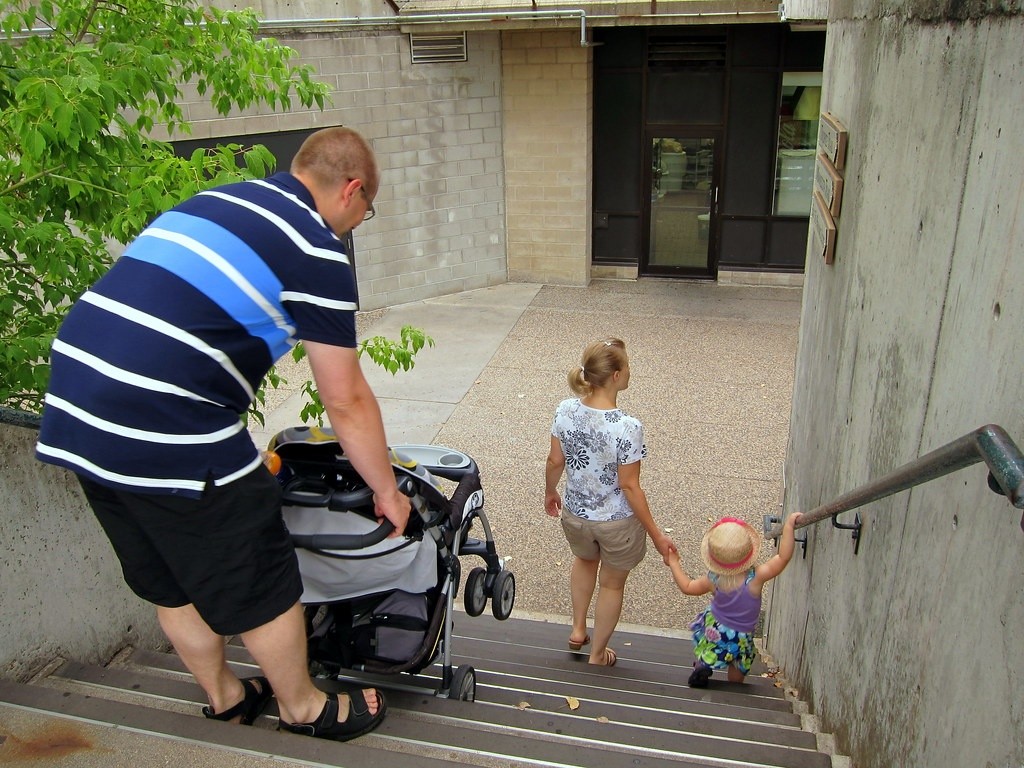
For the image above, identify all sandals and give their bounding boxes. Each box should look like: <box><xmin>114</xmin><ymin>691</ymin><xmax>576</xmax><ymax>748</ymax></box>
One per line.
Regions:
<box><xmin>202</xmin><ymin>677</ymin><xmax>275</xmax><ymax>726</ymax></box>
<box><xmin>276</xmin><ymin>687</ymin><xmax>389</xmax><ymax>742</ymax></box>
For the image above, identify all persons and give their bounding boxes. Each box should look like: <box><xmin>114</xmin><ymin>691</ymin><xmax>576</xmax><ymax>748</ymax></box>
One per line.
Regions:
<box><xmin>544</xmin><ymin>337</ymin><xmax>678</xmax><ymax>667</ymax></box>
<box><xmin>37</xmin><ymin>127</ymin><xmax>412</xmax><ymax>740</ymax></box>
<box><xmin>667</xmin><ymin>512</ymin><xmax>804</xmax><ymax>688</ymax></box>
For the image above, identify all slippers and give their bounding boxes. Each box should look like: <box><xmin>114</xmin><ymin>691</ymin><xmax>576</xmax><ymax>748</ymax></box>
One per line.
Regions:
<box><xmin>569</xmin><ymin>634</ymin><xmax>590</xmax><ymax>650</ymax></box>
<box><xmin>606</xmin><ymin>647</ymin><xmax>617</xmax><ymax>667</ymax></box>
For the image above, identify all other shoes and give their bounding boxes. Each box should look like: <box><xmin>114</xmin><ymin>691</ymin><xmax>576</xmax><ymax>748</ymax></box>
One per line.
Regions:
<box><xmin>688</xmin><ymin>662</ymin><xmax>713</xmax><ymax>689</ymax></box>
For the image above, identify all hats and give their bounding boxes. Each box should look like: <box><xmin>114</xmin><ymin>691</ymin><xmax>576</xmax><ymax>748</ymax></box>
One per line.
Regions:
<box><xmin>700</xmin><ymin>517</ymin><xmax>761</xmax><ymax>575</ymax></box>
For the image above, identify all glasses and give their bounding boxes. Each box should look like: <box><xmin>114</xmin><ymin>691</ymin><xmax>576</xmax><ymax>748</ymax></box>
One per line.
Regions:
<box><xmin>348</xmin><ymin>178</ymin><xmax>375</xmax><ymax>221</ymax></box>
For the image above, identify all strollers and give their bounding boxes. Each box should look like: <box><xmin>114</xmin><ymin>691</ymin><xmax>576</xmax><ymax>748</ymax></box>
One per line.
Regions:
<box><xmin>252</xmin><ymin>426</ymin><xmax>516</xmax><ymax>709</ymax></box>
